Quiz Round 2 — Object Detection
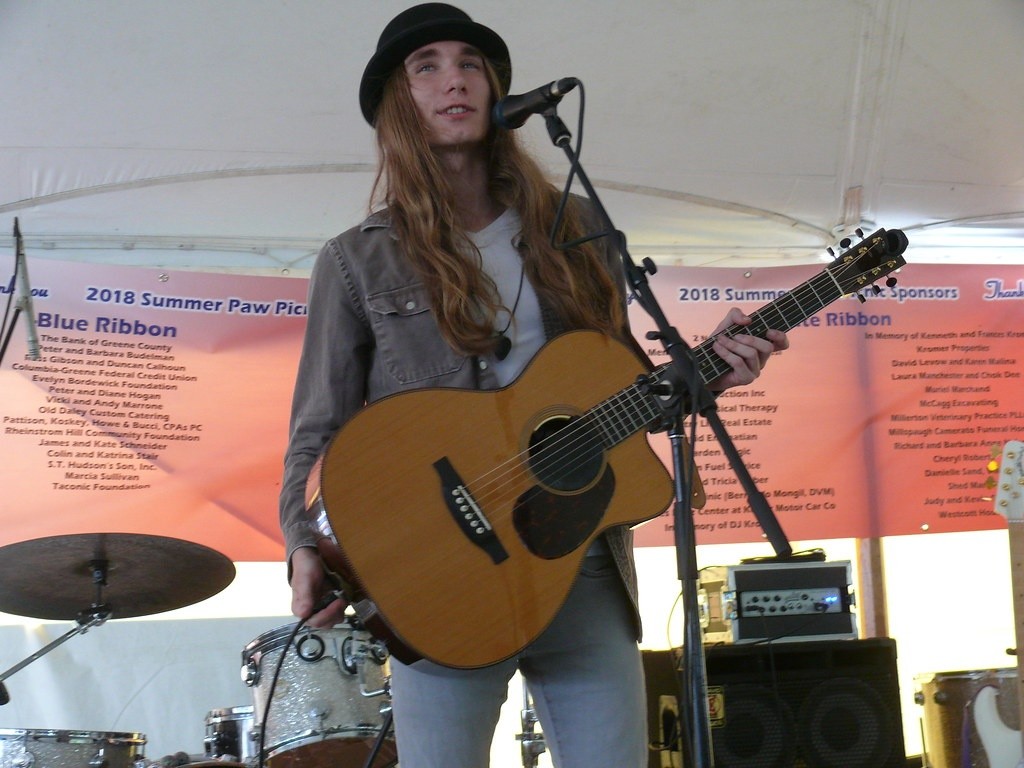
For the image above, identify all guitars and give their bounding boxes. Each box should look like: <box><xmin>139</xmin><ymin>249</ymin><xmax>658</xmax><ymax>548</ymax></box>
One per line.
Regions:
<box><xmin>303</xmin><ymin>227</ymin><xmax>909</xmax><ymax>678</ymax></box>
<box><xmin>966</xmin><ymin>439</ymin><xmax>1024</xmax><ymax>768</ymax></box>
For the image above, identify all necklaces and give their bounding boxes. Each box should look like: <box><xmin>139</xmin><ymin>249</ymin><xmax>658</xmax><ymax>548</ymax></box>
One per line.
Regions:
<box><xmin>465</xmin><ymin>262</ymin><xmax>525</xmax><ymax>361</ymax></box>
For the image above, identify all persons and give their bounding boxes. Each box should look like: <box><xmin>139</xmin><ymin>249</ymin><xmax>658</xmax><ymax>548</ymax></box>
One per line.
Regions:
<box><xmin>279</xmin><ymin>2</ymin><xmax>789</xmax><ymax>768</ymax></box>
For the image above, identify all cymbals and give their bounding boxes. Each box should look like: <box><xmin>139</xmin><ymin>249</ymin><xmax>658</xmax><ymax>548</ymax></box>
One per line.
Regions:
<box><xmin>0</xmin><ymin>532</ymin><xmax>236</xmax><ymax>623</ymax></box>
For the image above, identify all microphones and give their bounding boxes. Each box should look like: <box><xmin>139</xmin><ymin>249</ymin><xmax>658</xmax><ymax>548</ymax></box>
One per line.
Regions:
<box><xmin>13</xmin><ymin>225</ymin><xmax>40</xmax><ymax>361</ymax></box>
<box><xmin>492</xmin><ymin>76</ymin><xmax>578</xmax><ymax>130</ymax></box>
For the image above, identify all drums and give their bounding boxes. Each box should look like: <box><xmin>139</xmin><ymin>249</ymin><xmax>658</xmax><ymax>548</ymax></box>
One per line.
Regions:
<box><xmin>204</xmin><ymin>705</ymin><xmax>262</xmax><ymax>768</ymax></box>
<box><xmin>242</xmin><ymin>614</ymin><xmax>399</xmax><ymax>768</ymax></box>
<box><xmin>0</xmin><ymin>727</ymin><xmax>147</xmax><ymax>768</ymax></box>
<box><xmin>136</xmin><ymin>752</ymin><xmax>243</xmax><ymax>768</ymax></box>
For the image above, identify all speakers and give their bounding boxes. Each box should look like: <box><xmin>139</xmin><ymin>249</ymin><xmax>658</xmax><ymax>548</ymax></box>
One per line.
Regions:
<box><xmin>641</xmin><ymin>636</ymin><xmax>906</xmax><ymax>768</ymax></box>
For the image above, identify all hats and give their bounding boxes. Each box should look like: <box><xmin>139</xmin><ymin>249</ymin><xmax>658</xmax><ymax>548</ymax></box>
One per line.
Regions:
<box><xmin>358</xmin><ymin>3</ymin><xmax>513</xmax><ymax>129</ymax></box>
